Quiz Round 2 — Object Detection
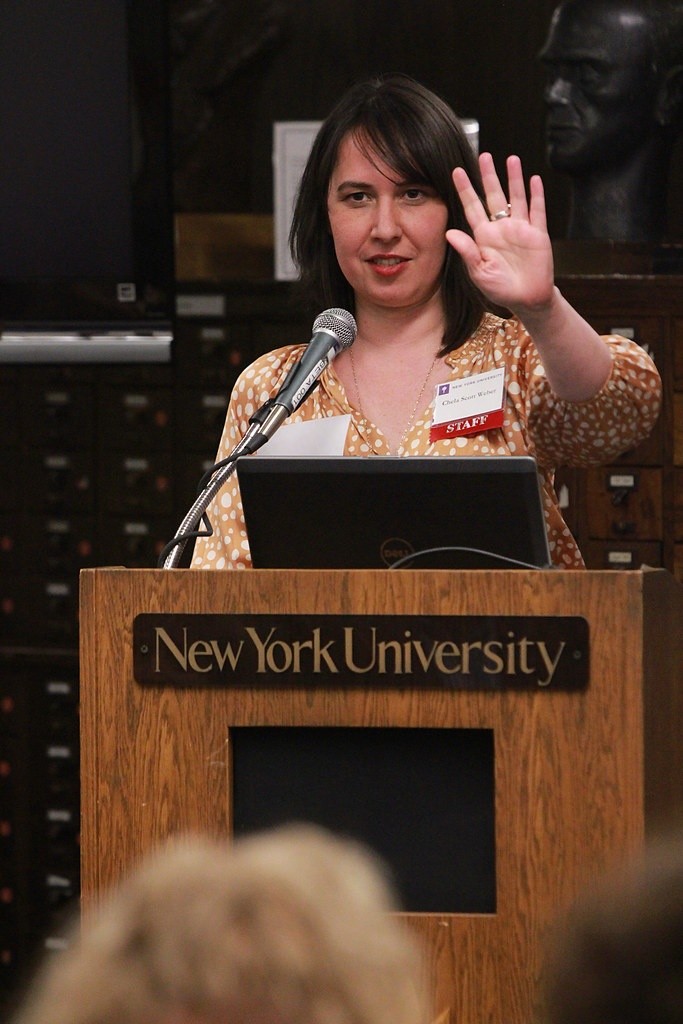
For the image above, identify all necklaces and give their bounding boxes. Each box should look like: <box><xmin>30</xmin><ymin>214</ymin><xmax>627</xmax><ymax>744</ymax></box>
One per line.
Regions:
<box><xmin>349</xmin><ymin>347</ymin><xmax>441</xmax><ymax>450</ymax></box>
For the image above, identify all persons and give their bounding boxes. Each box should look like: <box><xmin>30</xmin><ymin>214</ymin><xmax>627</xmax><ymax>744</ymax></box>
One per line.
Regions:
<box><xmin>540</xmin><ymin>0</ymin><xmax>683</xmax><ymax>238</ymax></box>
<box><xmin>190</xmin><ymin>75</ymin><xmax>663</xmax><ymax>569</ymax></box>
<box><xmin>24</xmin><ymin>825</ymin><xmax>432</xmax><ymax>1024</ymax></box>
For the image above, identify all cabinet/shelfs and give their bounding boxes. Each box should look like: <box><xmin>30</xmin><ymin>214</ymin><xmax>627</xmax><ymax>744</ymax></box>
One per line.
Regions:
<box><xmin>546</xmin><ymin>307</ymin><xmax>683</xmax><ymax>586</ymax></box>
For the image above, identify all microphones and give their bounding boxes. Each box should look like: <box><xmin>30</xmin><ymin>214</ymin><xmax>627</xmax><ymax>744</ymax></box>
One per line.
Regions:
<box><xmin>244</xmin><ymin>308</ymin><xmax>358</xmax><ymax>455</ymax></box>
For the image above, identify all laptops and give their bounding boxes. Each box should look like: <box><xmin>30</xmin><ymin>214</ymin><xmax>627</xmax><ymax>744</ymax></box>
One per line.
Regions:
<box><xmin>236</xmin><ymin>455</ymin><xmax>553</xmax><ymax>569</ymax></box>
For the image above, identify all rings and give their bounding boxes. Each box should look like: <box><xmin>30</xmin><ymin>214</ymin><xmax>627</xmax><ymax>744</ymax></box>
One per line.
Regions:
<box><xmin>490</xmin><ymin>210</ymin><xmax>510</xmax><ymax>220</ymax></box>
